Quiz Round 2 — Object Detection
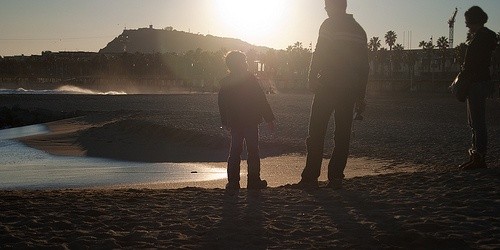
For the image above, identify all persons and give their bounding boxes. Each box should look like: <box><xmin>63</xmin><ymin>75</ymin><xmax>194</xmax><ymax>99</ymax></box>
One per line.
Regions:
<box><xmin>448</xmin><ymin>5</ymin><xmax>497</xmax><ymax>171</ymax></box>
<box><xmin>297</xmin><ymin>0</ymin><xmax>369</xmax><ymax>188</ymax></box>
<box><xmin>218</xmin><ymin>49</ymin><xmax>276</xmax><ymax>194</ymax></box>
